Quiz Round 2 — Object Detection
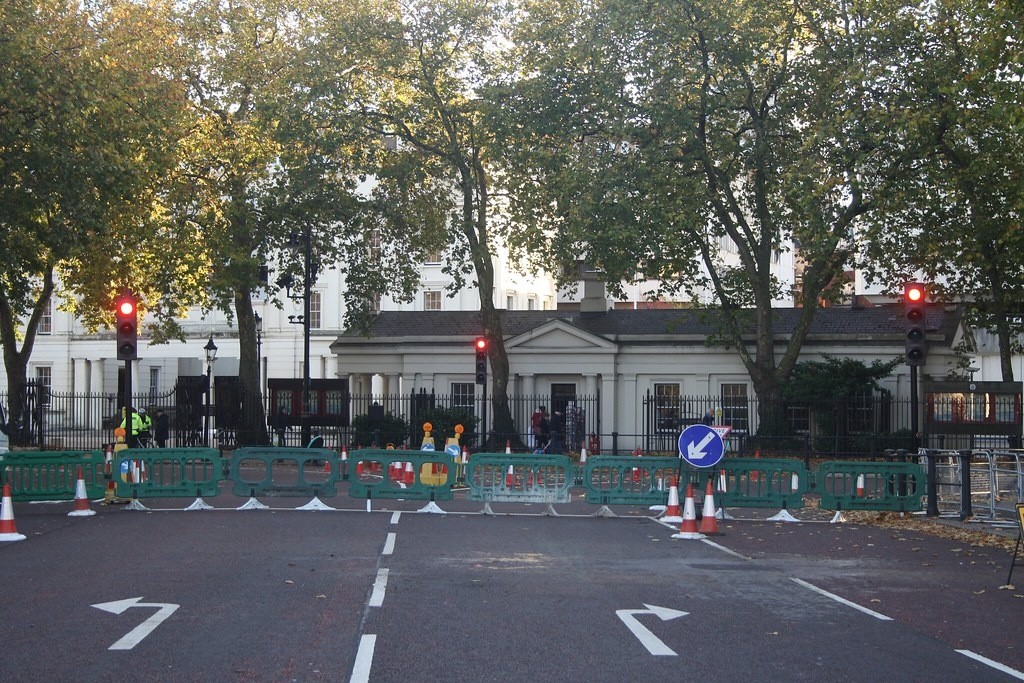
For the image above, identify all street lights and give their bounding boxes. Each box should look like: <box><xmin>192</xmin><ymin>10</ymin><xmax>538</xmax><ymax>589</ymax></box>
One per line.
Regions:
<box><xmin>203</xmin><ymin>334</ymin><xmax>218</xmax><ymax>447</ymax></box>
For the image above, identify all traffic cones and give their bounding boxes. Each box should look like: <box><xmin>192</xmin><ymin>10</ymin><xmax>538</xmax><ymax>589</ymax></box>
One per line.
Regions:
<box><xmin>0</xmin><ymin>443</ymin><xmax>884</xmax><ymax>543</ymax></box>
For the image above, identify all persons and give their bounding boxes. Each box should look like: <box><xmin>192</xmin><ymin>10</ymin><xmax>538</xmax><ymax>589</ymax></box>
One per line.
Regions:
<box><xmin>136</xmin><ymin>408</ymin><xmax>152</xmax><ymax>448</ymax></box>
<box><xmin>702</xmin><ymin>408</ymin><xmax>716</xmax><ymax>427</ymax></box>
<box><xmin>154</xmin><ymin>409</ymin><xmax>170</xmax><ymax>448</ymax></box>
<box><xmin>274</xmin><ymin>406</ymin><xmax>293</xmax><ymax>446</ymax></box>
<box><xmin>536</xmin><ymin>407</ymin><xmax>564</xmax><ymax>455</ymax></box>
<box><xmin>119</xmin><ymin>406</ymin><xmax>143</xmax><ymax>449</ymax></box>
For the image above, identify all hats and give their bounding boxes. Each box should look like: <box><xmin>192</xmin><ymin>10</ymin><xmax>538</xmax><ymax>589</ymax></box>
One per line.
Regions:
<box><xmin>138</xmin><ymin>408</ymin><xmax>146</xmax><ymax>414</ymax></box>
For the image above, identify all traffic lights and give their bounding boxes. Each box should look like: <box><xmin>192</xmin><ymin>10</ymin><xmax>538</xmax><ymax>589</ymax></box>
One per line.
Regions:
<box><xmin>904</xmin><ymin>283</ymin><xmax>927</xmax><ymax>367</ymax></box>
<box><xmin>474</xmin><ymin>337</ymin><xmax>488</xmax><ymax>385</ymax></box>
<box><xmin>115</xmin><ymin>297</ymin><xmax>137</xmax><ymax>360</ymax></box>
